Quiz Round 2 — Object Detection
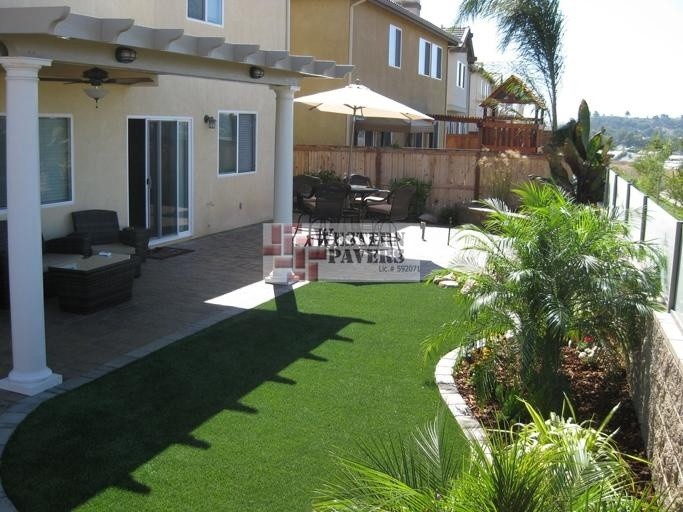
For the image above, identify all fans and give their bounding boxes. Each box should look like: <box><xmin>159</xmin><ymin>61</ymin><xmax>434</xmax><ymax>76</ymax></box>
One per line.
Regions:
<box><xmin>38</xmin><ymin>76</ymin><xmax>159</xmax><ymax>89</ymax></box>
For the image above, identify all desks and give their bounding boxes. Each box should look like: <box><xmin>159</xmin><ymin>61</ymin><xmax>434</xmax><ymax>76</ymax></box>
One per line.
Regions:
<box><xmin>339</xmin><ymin>184</ymin><xmax>391</xmax><ymax>236</ymax></box>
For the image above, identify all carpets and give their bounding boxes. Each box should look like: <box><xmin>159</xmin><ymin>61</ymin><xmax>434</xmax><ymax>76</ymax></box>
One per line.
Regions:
<box><xmin>147</xmin><ymin>246</ymin><xmax>196</xmax><ymax>260</ymax></box>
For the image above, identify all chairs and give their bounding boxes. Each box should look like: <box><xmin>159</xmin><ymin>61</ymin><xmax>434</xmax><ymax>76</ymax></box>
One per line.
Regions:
<box><xmin>343</xmin><ymin>173</ymin><xmax>375</xmax><ymax>225</ymax></box>
<box><xmin>359</xmin><ymin>184</ymin><xmax>417</xmax><ymax>256</ymax></box>
<box><xmin>294</xmin><ymin>174</ymin><xmax>322</xmax><ymax>221</ymax></box>
<box><xmin>291</xmin><ymin>182</ymin><xmax>351</xmax><ymax>247</ymax></box>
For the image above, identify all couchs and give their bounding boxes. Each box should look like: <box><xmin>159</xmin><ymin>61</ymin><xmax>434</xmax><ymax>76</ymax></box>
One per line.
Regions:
<box><xmin>1</xmin><ymin>220</ymin><xmax>85</xmax><ymax>306</ymax></box>
<box><xmin>71</xmin><ymin>209</ymin><xmax>150</xmax><ymax>279</ymax></box>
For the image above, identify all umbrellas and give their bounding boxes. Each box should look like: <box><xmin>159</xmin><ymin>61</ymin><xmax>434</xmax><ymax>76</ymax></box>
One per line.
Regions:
<box><xmin>294</xmin><ymin>79</ymin><xmax>435</xmax><ymax>186</ymax></box>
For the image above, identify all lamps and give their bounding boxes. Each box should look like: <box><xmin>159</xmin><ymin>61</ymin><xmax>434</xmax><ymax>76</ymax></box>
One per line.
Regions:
<box><xmin>81</xmin><ymin>67</ymin><xmax>110</xmax><ymax>109</ymax></box>
<box><xmin>204</xmin><ymin>115</ymin><xmax>216</xmax><ymax>129</ymax></box>
